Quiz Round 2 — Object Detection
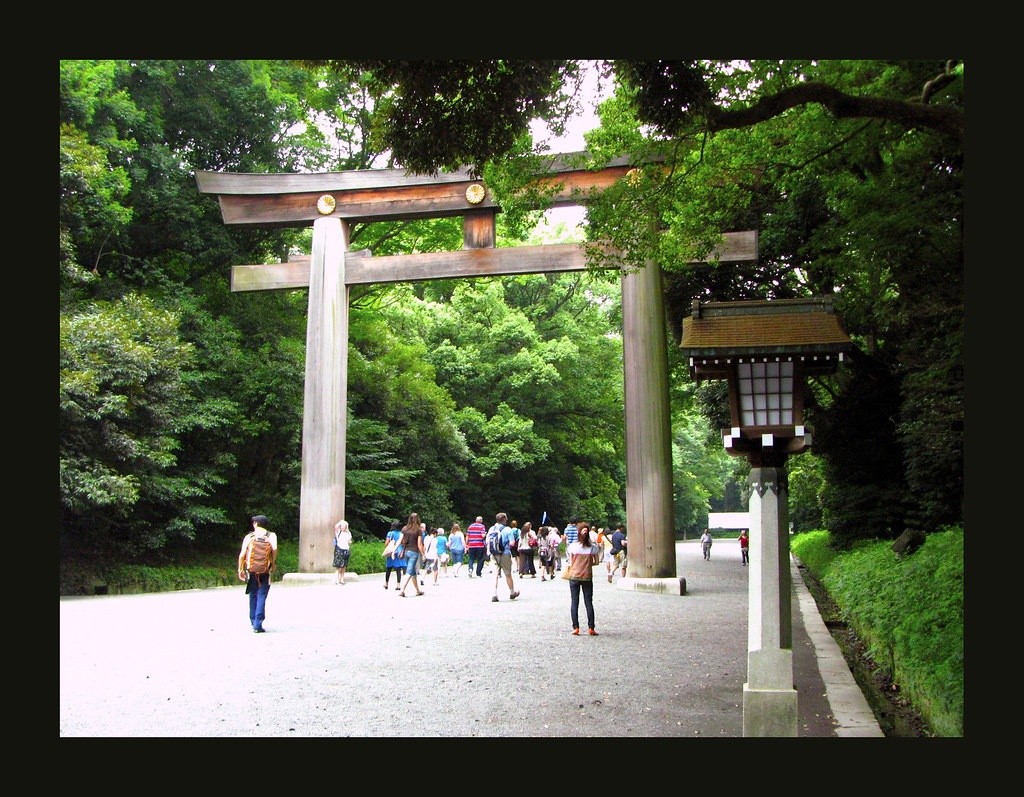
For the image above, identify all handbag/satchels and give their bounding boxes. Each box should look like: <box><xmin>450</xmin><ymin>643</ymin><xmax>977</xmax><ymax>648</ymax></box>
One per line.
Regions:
<box><xmin>538</xmin><ymin>549</ymin><xmax>548</xmax><ymax>557</ymax></box>
<box><xmin>398</xmin><ymin>550</ymin><xmax>404</xmax><ymax>558</ymax></box>
<box><xmin>334</xmin><ymin>531</ymin><xmax>341</xmax><ymax>546</ymax></box>
<box><xmin>382</xmin><ymin>537</ymin><xmax>395</xmax><ymax>556</ymax></box>
<box><xmin>513</xmin><ymin>528</ymin><xmax>519</xmax><ymax>548</ymax></box>
<box><xmin>610</xmin><ymin>547</ymin><xmax>619</xmax><ymax>555</ymax></box>
<box><xmin>529</xmin><ymin>537</ymin><xmax>538</xmax><ymax>546</ymax></box>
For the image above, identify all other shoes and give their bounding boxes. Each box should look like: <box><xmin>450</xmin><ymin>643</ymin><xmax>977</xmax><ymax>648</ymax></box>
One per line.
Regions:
<box><xmin>255</xmin><ymin>628</ymin><xmax>265</xmax><ymax>632</ymax></box>
<box><xmin>402</xmin><ymin>593</ymin><xmax>405</xmax><ymax>596</ymax></box>
<box><xmin>416</xmin><ymin>591</ymin><xmax>424</xmax><ymax>596</ymax></box>
<box><xmin>551</xmin><ymin>576</ymin><xmax>555</xmax><ymax>579</ymax></box>
<box><xmin>510</xmin><ymin>591</ymin><xmax>520</xmax><ymax>599</ymax></box>
<box><xmin>492</xmin><ymin>596</ymin><xmax>499</xmax><ymax>601</ymax></box>
<box><xmin>542</xmin><ymin>578</ymin><xmax>546</xmax><ymax>581</ymax></box>
<box><xmin>396</xmin><ymin>587</ymin><xmax>400</xmax><ymax>590</ymax></box>
<box><xmin>383</xmin><ymin>585</ymin><xmax>388</xmax><ymax>589</ymax></box>
<box><xmin>572</xmin><ymin>630</ymin><xmax>579</xmax><ymax>635</ymax></box>
<box><xmin>590</xmin><ymin>630</ymin><xmax>599</xmax><ymax>635</ymax></box>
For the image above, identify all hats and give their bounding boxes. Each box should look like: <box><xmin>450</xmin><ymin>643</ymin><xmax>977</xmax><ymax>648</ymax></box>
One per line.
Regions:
<box><xmin>597</xmin><ymin>528</ymin><xmax>604</xmax><ymax>534</ymax></box>
<box><xmin>252</xmin><ymin>515</ymin><xmax>267</xmax><ymax>524</ymax></box>
<box><xmin>569</xmin><ymin>516</ymin><xmax>579</xmax><ymax>522</ymax></box>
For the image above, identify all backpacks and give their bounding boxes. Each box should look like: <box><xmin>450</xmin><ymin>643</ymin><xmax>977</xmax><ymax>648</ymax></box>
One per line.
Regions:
<box><xmin>246</xmin><ymin>532</ymin><xmax>272</xmax><ymax>574</ymax></box>
<box><xmin>487</xmin><ymin>525</ymin><xmax>505</xmax><ymax>554</ymax></box>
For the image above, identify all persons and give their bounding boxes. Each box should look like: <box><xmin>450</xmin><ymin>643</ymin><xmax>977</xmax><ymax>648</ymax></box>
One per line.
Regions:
<box><xmin>565</xmin><ymin>524</ymin><xmax>599</xmax><ymax>636</ymax></box>
<box><xmin>700</xmin><ymin>529</ymin><xmax>713</xmax><ymax>561</ymax></box>
<box><xmin>333</xmin><ymin>521</ymin><xmax>352</xmax><ymax>585</ymax></box>
<box><xmin>239</xmin><ymin>515</ymin><xmax>278</xmax><ymax>634</ymax></box>
<box><xmin>737</xmin><ymin>531</ymin><xmax>749</xmax><ymax>566</ymax></box>
<box><xmin>383</xmin><ymin>514</ymin><xmax>629</xmax><ymax>601</ymax></box>
